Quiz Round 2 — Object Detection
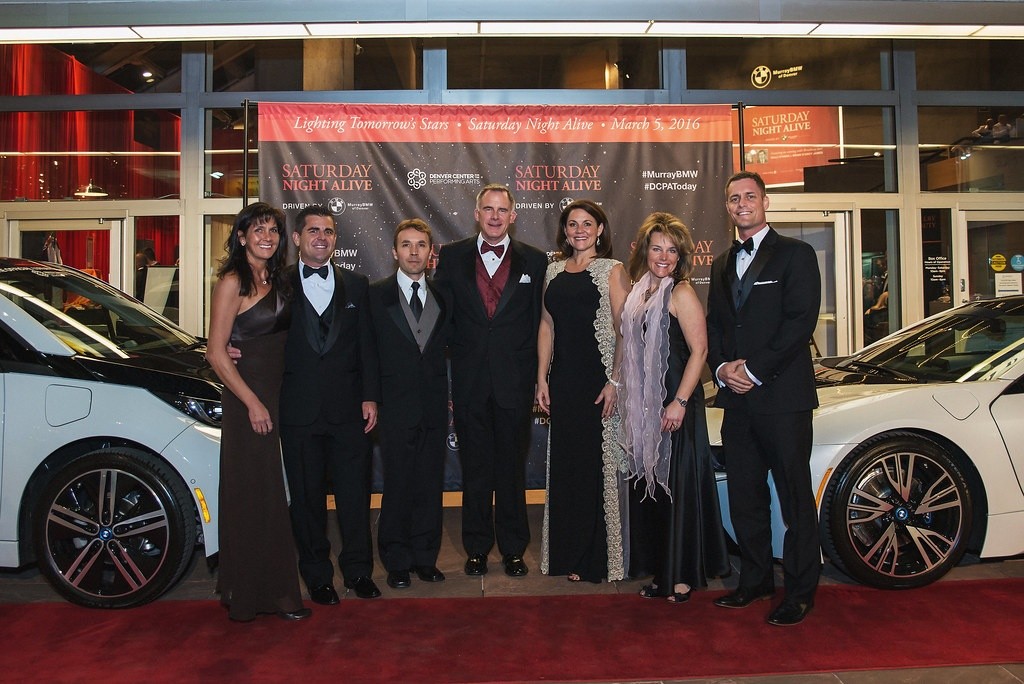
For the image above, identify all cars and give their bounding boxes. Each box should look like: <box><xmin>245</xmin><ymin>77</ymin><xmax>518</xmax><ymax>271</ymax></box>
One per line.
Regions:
<box><xmin>703</xmin><ymin>295</ymin><xmax>1024</xmax><ymax>595</ymax></box>
<box><xmin>0</xmin><ymin>257</ymin><xmax>232</xmax><ymax>610</ymax></box>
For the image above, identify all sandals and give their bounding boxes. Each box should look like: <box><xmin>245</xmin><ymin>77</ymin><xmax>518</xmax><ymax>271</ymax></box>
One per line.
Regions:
<box><xmin>667</xmin><ymin>584</ymin><xmax>693</xmax><ymax>603</ymax></box>
<box><xmin>567</xmin><ymin>573</ymin><xmax>589</xmax><ymax>581</ymax></box>
<box><xmin>640</xmin><ymin>582</ymin><xmax>665</xmax><ymax>599</ymax></box>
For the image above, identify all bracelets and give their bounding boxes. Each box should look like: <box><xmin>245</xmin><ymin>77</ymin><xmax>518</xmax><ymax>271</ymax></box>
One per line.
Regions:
<box><xmin>608</xmin><ymin>377</ymin><xmax>620</xmax><ymax>386</ymax></box>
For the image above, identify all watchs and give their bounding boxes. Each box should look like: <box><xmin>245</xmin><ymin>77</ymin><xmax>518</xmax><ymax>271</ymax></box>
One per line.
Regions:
<box><xmin>672</xmin><ymin>396</ymin><xmax>687</xmax><ymax>407</ymax></box>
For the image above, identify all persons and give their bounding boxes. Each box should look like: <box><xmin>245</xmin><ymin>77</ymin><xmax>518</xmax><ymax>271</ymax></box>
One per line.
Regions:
<box><xmin>862</xmin><ymin>257</ymin><xmax>890</xmax><ymax>347</ymax></box>
<box><xmin>23</xmin><ymin>231</ymin><xmax>62</xmax><ymax>265</ymax></box>
<box><xmin>745</xmin><ymin>154</ymin><xmax>754</xmax><ymax>164</ymax></box>
<box><xmin>757</xmin><ymin>150</ymin><xmax>767</xmax><ymax>163</ymax></box>
<box><xmin>143</xmin><ymin>247</ymin><xmax>161</xmax><ymax>266</ymax></box>
<box><xmin>706</xmin><ymin>169</ymin><xmax>822</xmax><ymax>626</ymax></box>
<box><xmin>135</xmin><ymin>252</ymin><xmax>147</xmax><ymax>302</ymax></box>
<box><xmin>207</xmin><ymin>202</ymin><xmax>313</xmax><ymax>624</ymax></box>
<box><xmin>619</xmin><ymin>213</ymin><xmax>709</xmax><ymax>605</ymax></box>
<box><xmin>223</xmin><ymin>205</ymin><xmax>384</xmax><ymax>605</ymax></box>
<box><xmin>432</xmin><ymin>184</ymin><xmax>549</xmax><ymax>578</ymax></box>
<box><xmin>367</xmin><ymin>218</ymin><xmax>454</xmax><ymax>588</ymax></box>
<box><xmin>536</xmin><ymin>199</ymin><xmax>634</xmax><ymax>582</ymax></box>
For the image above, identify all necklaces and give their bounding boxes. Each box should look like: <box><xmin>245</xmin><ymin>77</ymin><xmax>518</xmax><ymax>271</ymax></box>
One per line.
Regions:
<box><xmin>250</xmin><ymin>265</ymin><xmax>267</xmax><ymax>285</ymax></box>
<box><xmin>643</xmin><ymin>285</ymin><xmax>659</xmax><ymax>302</ymax></box>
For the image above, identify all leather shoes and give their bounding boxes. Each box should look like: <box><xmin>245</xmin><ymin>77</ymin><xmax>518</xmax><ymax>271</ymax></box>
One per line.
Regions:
<box><xmin>308</xmin><ymin>584</ymin><xmax>339</xmax><ymax>604</ymax></box>
<box><xmin>387</xmin><ymin>570</ymin><xmax>411</xmax><ymax>588</ymax></box>
<box><xmin>713</xmin><ymin>587</ymin><xmax>775</xmax><ymax>608</ymax></box>
<box><xmin>465</xmin><ymin>553</ymin><xmax>488</xmax><ymax>575</ymax></box>
<box><xmin>766</xmin><ymin>596</ymin><xmax>814</xmax><ymax>626</ymax></box>
<box><xmin>503</xmin><ymin>554</ymin><xmax>528</xmax><ymax>576</ymax></box>
<box><xmin>278</xmin><ymin>608</ymin><xmax>312</xmax><ymax>621</ymax></box>
<box><xmin>344</xmin><ymin>575</ymin><xmax>381</xmax><ymax>598</ymax></box>
<box><xmin>409</xmin><ymin>564</ymin><xmax>445</xmax><ymax>582</ymax></box>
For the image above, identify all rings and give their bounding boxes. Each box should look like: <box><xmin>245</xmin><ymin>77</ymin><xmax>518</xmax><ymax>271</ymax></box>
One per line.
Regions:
<box><xmin>613</xmin><ymin>405</ymin><xmax>616</xmax><ymax>409</ymax></box>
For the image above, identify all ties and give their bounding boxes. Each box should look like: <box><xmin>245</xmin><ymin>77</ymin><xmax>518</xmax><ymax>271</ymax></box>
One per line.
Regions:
<box><xmin>409</xmin><ymin>282</ymin><xmax>423</xmax><ymax>323</ymax></box>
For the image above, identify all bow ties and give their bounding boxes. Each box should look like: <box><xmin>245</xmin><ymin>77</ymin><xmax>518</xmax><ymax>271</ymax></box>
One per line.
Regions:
<box><xmin>303</xmin><ymin>265</ymin><xmax>328</xmax><ymax>280</ymax></box>
<box><xmin>481</xmin><ymin>240</ymin><xmax>504</xmax><ymax>259</ymax></box>
<box><xmin>731</xmin><ymin>237</ymin><xmax>754</xmax><ymax>257</ymax></box>
